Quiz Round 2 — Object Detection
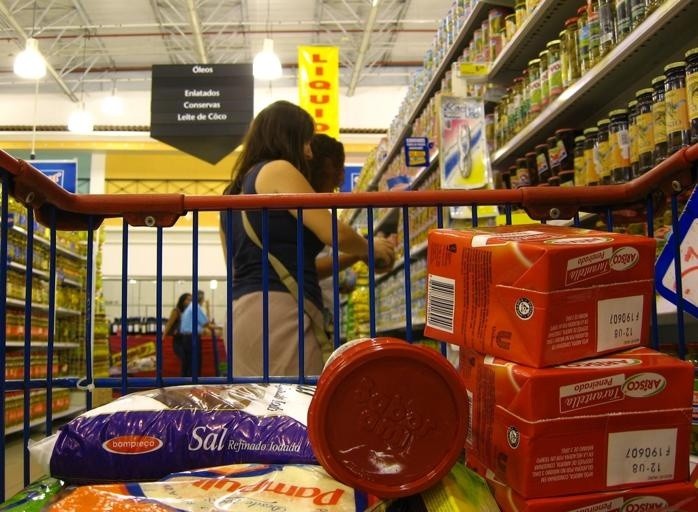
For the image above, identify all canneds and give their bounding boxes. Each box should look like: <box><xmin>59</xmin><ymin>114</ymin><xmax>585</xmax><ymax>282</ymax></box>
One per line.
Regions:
<box><xmin>512</xmin><ymin>77</ymin><xmax>525</xmax><ymax>131</ymax></box>
<box><xmin>527</xmin><ymin>58</ymin><xmax>541</xmax><ymax>113</ymax></box>
<box><xmin>514</xmin><ymin>1</ymin><xmax>526</xmax><ymax>27</ymax></box>
<box><xmin>577</xmin><ymin>6</ymin><xmax>589</xmax><ymax>71</ymax></box>
<box><xmin>607</xmin><ymin>110</ymin><xmax>631</xmax><ymax>184</ymax></box>
<box><xmin>555</xmin><ymin>128</ymin><xmax>577</xmax><ymax>169</ymax></box>
<box><xmin>627</xmin><ymin>100</ymin><xmax>641</xmax><ymax>178</ymax></box>
<box><xmin>547</xmin><ymin>138</ymin><xmax>560</xmax><ymax>174</ymax></box>
<box><xmin>613</xmin><ymin>0</ymin><xmax>630</xmax><ymax>43</ymax></box>
<box><xmin>564</xmin><ymin>17</ymin><xmax>579</xmax><ymax>80</ymax></box>
<box><xmin>636</xmin><ymin>87</ymin><xmax>655</xmax><ymax>173</ymax></box>
<box><xmin>525</xmin><ymin>151</ymin><xmax>537</xmax><ymax>184</ymax></box>
<box><xmin>4</xmin><ymin>195</ymin><xmax>71</xmax><ymax>426</ymax></box>
<box><xmin>521</xmin><ymin>69</ymin><xmax>531</xmax><ymax>123</ymax></box>
<box><xmin>597</xmin><ymin>120</ymin><xmax>610</xmax><ymax>185</ymax></box>
<box><xmin>546</xmin><ymin>39</ymin><xmax>563</xmax><ymax>100</ymax></box>
<box><xmin>525</xmin><ymin>0</ymin><xmax>538</xmax><ymax>13</ymax></box>
<box><xmin>509</xmin><ymin>166</ymin><xmax>518</xmax><ymax>189</ymax></box>
<box><xmin>646</xmin><ymin>1</ymin><xmax>665</xmax><ymax>10</ymax></box>
<box><xmin>557</xmin><ymin>169</ymin><xmax>576</xmax><ymax>188</ymax></box>
<box><xmin>583</xmin><ymin>128</ymin><xmax>602</xmax><ymax>186</ymax></box>
<box><xmin>490</xmin><ymin>8</ymin><xmax>501</xmax><ymax>39</ymax></box>
<box><xmin>535</xmin><ymin>144</ymin><xmax>551</xmax><ymax>179</ymax></box>
<box><xmin>537</xmin><ymin>182</ymin><xmax>550</xmax><ymax>188</ymax></box>
<box><xmin>538</xmin><ymin>49</ymin><xmax>551</xmax><ymax>107</ymax></box>
<box><xmin>504</xmin><ymin>14</ymin><xmax>516</xmax><ymax>39</ymax></box>
<box><xmin>501</xmin><ymin>172</ymin><xmax>510</xmax><ymax>189</ymax></box>
<box><xmin>483</xmin><ymin>85</ymin><xmax>514</xmax><ymax>154</ymax></box>
<box><xmin>650</xmin><ymin>74</ymin><xmax>669</xmax><ymax>164</ymax></box>
<box><xmin>587</xmin><ymin>0</ymin><xmax>600</xmax><ymax>66</ymax></box>
<box><xmin>662</xmin><ymin>62</ymin><xmax>687</xmax><ymax>153</ymax></box>
<box><xmin>546</xmin><ymin>176</ymin><xmax>559</xmax><ymax>189</ymax></box>
<box><xmin>481</xmin><ymin>20</ymin><xmax>493</xmax><ymax>49</ymax></box>
<box><xmin>572</xmin><ymin>134</ymin><xmax>586</xmax><ymax>187</ymax></box>
<box><xmin>499</xmin><ymin>28</ymin><xmax>508</xmax><ymax>48</ymax></box>
<box><xmin>111</xmin><ymin>317</ymin><xmax>171</xmax><ymax>334</ymax></box>
<box><xmin>631</xmin><ymin>0</ymin><xmax>645</xmax><ymax>29</ymax></box>
<box><xmin>515</xmin><ymin>158</ymin><xmax>529</xmax><ymax>187</ymax></box>
<box><xmin>684</xmin><ymin>48</ymin><xmax>698</xmax><ymax>144</ymax></box>
<box><xmin>558</xmin><ymin>28</ymin><xmax>570</xmax><ymax>89</ymax></box>
<box><xmin>598</xmin><ymin>0</ymin><xmax>612</xmax><ymax>57</ymax></box>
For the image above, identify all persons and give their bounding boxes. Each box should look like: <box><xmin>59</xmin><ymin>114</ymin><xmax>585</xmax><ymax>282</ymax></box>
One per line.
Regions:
<box><xmin>181</xmin><ymin>291</ymin><xmax>214</xmax><ymax>377</ymax></box>
<box><xmin>292</xmin><ymin>134</ymin><xmax>345</xmax><ymax>364</ymax></box>
<box><xmin>223</xmin><ymin>100</ymin><xmax>396</xmax><ymax>383</ymax></box>
<box><xmin>161</xmin><ymin>293</ymin><xmax>189</xmax><ymax>377</ymax></box>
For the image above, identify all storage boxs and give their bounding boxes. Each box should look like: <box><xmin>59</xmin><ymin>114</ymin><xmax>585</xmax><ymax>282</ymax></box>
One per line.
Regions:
<box><xmin>424</xmin><ymin>223</ymin><xmax>658</xmax><ymax>365</ymax></box>
<box><xmin>452</xmin><ymin>344</ymin><xmax>698</xmax><ymax>493</ymax></box>
<box><xmin>461</xmin><ymin>450</ymin><xmax>698</xmax><ymax>508</ymax></box>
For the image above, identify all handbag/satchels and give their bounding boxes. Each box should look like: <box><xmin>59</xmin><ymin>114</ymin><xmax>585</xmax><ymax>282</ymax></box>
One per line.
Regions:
<box><xmin>236</xmin><ymin>175</ymin><xmax>337</xmax><ymax>353</ymax></box>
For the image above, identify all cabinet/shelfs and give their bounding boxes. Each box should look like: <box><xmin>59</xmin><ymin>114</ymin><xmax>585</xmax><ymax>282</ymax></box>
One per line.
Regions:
<box><xmin>319</xmin><ymin>0</ymin><xmax>698</xmax><ymax>454</ymax></box>
<box><xmin>1</xmin><ymin>181</ymin><xmax>98</xmax><ymax>443</ymax></box>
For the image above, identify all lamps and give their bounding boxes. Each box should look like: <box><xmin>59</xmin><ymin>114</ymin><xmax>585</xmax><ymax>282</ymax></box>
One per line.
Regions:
<box><xmin>252</xmin><ymin>0</ymin><xmax>282</xmax><ymax>82</ymax></box>
<box><xmin>66</xmin><ymin>30</ymin><xmax>94</xmax><ymax>133</ymax></box>
<box><xmin>12</xmin><ymin>1</ymin><xmax>48</xmax><ymax>81</ymax></box>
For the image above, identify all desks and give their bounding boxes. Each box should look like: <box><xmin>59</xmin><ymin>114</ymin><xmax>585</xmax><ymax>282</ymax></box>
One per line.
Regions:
<box><xmin>110</xmin><ymin>333</ymin><xmax>226</xmax><ymax>394</ymax></box>
